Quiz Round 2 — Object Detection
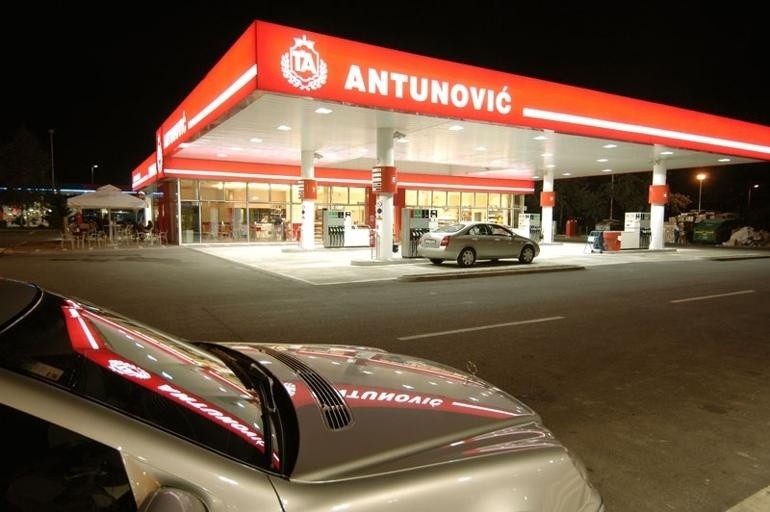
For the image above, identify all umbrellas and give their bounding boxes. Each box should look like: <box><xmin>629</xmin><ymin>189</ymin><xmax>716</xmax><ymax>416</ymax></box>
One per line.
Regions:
<box><xmin>66</xmin><ymin>183</ymin><xmax>147</xmax><ymax>242</ymax></box>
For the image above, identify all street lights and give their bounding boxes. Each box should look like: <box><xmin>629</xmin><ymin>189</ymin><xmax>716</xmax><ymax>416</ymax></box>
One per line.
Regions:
<box><xmin>697</xmin><ymin>172</ymin><xmax>706</xmax><ymax>212</ymax></box>
<box><xmin>747</xmin><ymin>184</ymin><xmax>759</xmax><ymax>225</ymax></box>
<box><xmin>90</xmin><ymin>164</ymin><xmax>98</xmax><ymax>189</ymax></box>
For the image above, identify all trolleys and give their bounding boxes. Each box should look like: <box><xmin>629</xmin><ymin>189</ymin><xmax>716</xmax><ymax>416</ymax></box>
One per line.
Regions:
<box><xmin>584</xmin><ymin>227</ymin><xmax>604</xmax><ymax>253</ymax></box>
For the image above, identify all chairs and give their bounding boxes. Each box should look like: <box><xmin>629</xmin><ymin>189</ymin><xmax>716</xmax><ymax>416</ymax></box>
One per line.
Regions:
<box><xmin>60</xmin><ymin>225</ymin><xmax>169</xmax><ymax>249</ymax></box>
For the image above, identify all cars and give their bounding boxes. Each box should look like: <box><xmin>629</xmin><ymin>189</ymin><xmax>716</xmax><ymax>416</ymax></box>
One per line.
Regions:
<box><xmin>0</xmin><ymin>275</ymin><xmax>608</xmax><ymax>511</ymax></box>
<box><xmin>417</xmin><ymin>220</ymin><xmax>540</xmax><ymax>267</ymax></box>
